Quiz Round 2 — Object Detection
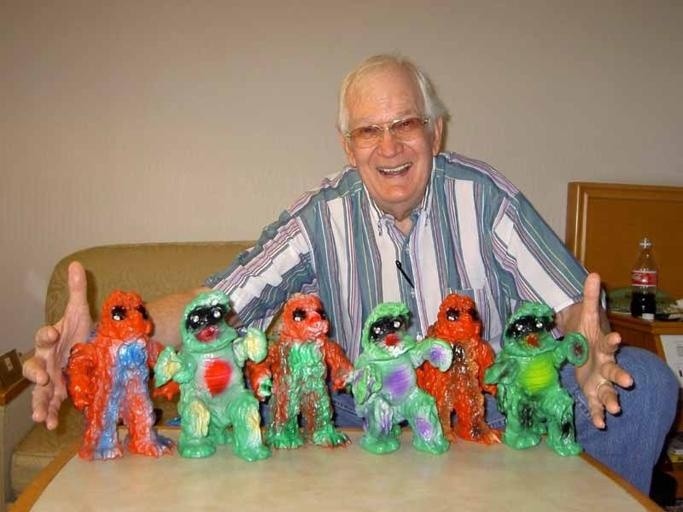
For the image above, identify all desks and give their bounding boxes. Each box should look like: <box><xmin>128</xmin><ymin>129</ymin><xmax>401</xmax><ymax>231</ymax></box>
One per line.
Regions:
<box><xmin>12</xmin><ymin>425</ymin><xmax>667</xmax><ymax>511</ymax></box>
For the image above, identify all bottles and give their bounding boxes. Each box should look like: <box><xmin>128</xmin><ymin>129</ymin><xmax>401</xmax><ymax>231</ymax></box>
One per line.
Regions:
<box><xmin>631</xmin><ymin>236</ymin><xmax>659</xmax><ymax>318</ymax></box>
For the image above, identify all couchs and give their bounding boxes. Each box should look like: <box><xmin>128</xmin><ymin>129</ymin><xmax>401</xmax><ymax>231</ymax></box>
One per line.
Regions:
<box><xmin>0</xmin><ymin>240</ymin><xmax>260</xmax><ymax>496</ymax></box>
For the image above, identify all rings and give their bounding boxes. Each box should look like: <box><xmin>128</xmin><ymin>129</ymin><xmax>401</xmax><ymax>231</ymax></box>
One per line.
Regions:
<box><xmin>592</xmin><ymin>382</ymin><xmax>610</xmax><ymax>394</ymax></box>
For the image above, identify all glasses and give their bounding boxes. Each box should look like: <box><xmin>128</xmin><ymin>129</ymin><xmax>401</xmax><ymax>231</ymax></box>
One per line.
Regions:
<box><xmin>342</xmin><ymin>116</ymin><xmax>428</xmax><ymax>148</ymax></box>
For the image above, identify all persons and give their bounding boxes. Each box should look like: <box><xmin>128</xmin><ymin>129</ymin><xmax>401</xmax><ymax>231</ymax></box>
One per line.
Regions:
<box><xmin>18</xmin><ymin>52</ymin><xmax>679</xmax><ymax>499</ymax></box>
